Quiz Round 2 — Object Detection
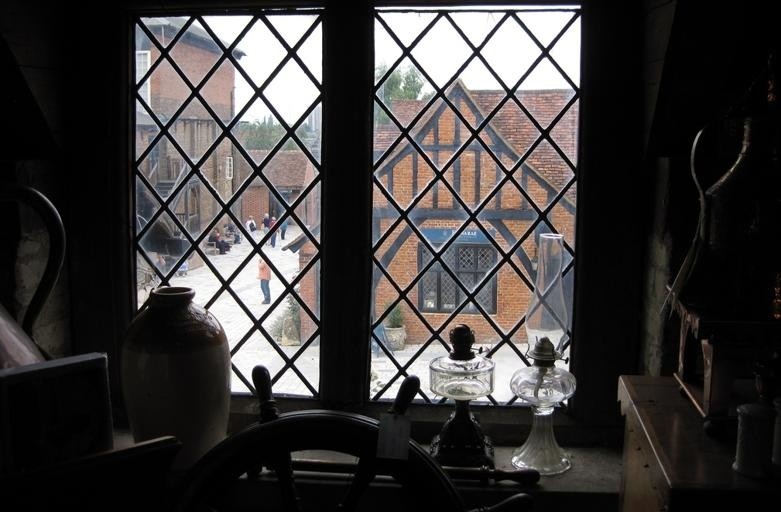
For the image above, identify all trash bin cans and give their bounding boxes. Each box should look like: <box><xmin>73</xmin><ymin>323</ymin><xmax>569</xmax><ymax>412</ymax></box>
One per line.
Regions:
<box><xmin>371</xmin><ymin>325</ymin><xmax>383</xmax><ymax>351</ymax></box>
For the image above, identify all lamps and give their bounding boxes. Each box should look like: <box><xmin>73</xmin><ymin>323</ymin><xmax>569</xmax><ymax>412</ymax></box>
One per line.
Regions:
<box><xmin>509</xmin><ymin>235</ymin><xmax>578</xmax><ymax>477</ymax></box>
<box><xmin>429</xmin><ymin>323</ymin><xmax>495</xmax><ymax>475</ymax></box>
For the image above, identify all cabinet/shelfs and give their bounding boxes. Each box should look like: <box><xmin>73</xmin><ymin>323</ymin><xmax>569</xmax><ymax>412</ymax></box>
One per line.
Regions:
<box><xmin>614</xmin><ymin>378</ymin><xmax>780</xmax><ymax>511</ymax></box>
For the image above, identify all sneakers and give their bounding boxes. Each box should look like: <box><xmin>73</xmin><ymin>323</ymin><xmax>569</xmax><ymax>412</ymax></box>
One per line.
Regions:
<box><xmin>261</xmin><ymin>300</ymin><xmax>270</xmax><ymax>304</ymax></box>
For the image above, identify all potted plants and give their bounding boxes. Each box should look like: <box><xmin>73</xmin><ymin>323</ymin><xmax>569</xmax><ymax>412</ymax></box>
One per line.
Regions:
<box><xmin>382</xmin><ymin>300</ymin><xmax>407</xmax><ymax>352</ymax></box>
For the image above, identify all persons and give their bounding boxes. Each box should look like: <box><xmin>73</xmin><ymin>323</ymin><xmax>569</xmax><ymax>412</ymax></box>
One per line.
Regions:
<box><xmin>207</xmin><ymin>208</ymin><xmax>288</xmax><ymax>255</ymax></box>
<box><xmin>256</xmin><ymin>256</ymin><xmax>271</xmax><ymax>304</ymax></box>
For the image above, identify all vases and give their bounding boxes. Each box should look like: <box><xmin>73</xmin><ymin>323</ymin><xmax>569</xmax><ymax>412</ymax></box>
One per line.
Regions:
<box><xmin>120</xmin><ymin>286</ymin><xmax>231</xmax><ymax>477</ymax></box>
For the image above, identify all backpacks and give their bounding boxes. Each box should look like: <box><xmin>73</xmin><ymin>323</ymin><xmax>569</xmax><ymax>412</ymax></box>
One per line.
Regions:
<box><xmin>249</xmin><ymin>220</ymin><xmax>256</xmax><ymax>231</ymax></box>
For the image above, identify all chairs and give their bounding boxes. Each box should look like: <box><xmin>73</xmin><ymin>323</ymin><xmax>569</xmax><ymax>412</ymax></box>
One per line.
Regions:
<box><xmin>208</xmin><ymin>223</ymin><xmax>242</xmax><ymax>254</ymax></box>
